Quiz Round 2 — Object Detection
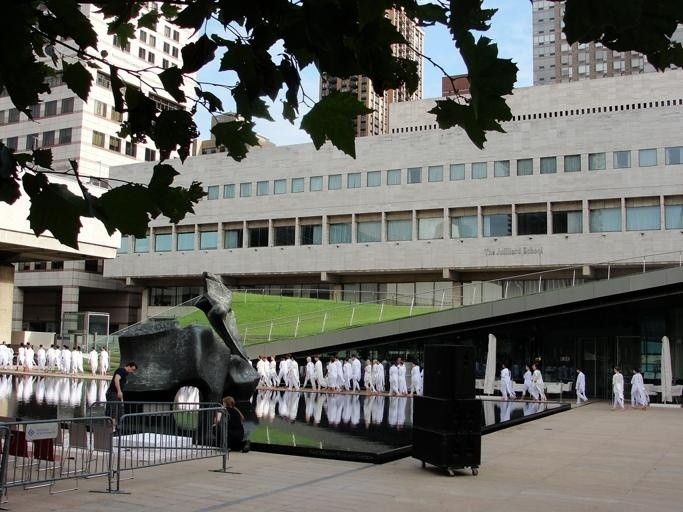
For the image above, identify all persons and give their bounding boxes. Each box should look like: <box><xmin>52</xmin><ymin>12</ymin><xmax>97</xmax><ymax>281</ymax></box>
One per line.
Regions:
<box><xmin>630</xmin><ymin>367</ymin><xmax>650</xmax><ymax>406</ymax></box>
<box><xmin>255</xmin><ymin>352</ymin><xmax>423</xmax><ymax>395</ymax></box>
<box><xmin>610</xmin><ymin>366</ymin><xmax>627</xmax><ymax>412</ymax></box>
<box><xmin>499</xmin><ymin>362</ymin><xmax>517</xmax><ymax>400</ymax></box>
<box><xmin>1</xmin><ymin>341</ymin><xmax>108</xmax><ymax>376</ymax></box>
<box><xmin>574</xmin><ymin>366</ymin><xmax>588</xmax><ymax>405</ymax></box>
<box><xmin>529</xmin><ymin>362</ymin><xmax>547</xmax><ymax>400</ymax></box>
<box><xmin>209</xmin><ymin>396</ymin><xmax>252</xmax><ymax>453</ymax></box>
<box><xmin>254</xmin><ymin>388</ymin><xmax>414</xmax><ymax>430</ymax></box>
<box><xmin>0</xmin><ymin>372</ymin><xmax>108</xmax><ymax>409</ymax></box>
<box><xmin>175</xmin><ymin>386</ymin><xmax>201</xmax><ymax>415</ymax></box>
<box><xmin>518</xmin><ymin>362</ymin><xmax>535</xmax><ymax>399</ymax></box>
<box><xmin>103</xmin><ymin>360</ymin><xmax>137</xmax><ymax>435</ymax></box>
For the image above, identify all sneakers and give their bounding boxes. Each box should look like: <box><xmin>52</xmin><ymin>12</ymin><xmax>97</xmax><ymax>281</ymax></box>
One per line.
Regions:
<box><xmin>243</xmin><ymin>440</ymin><xmax>249</xmax><ymax>452</ymax></box>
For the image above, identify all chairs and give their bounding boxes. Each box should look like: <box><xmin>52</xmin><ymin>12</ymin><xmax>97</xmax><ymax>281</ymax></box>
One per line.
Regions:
<box><xmin>0</xmin><ymin>416</ymin><xmax>136</xmax><ymax>495</ymax></box>
<box><xmin>475</xmin><ymin>378</ymin><xmax>573</xmax><ymax>403</ymax></box>
<box><xmin>644</xmin><ymin>384</ymin><xmax>683</xmax><ymax>408</ymax></box>
